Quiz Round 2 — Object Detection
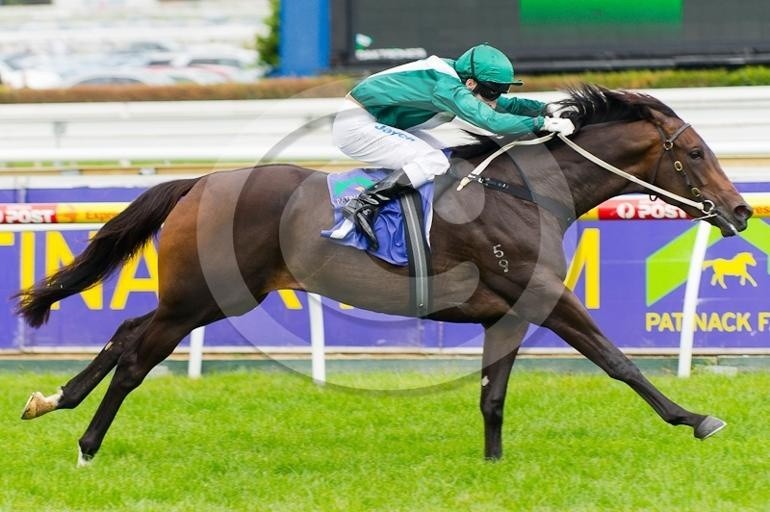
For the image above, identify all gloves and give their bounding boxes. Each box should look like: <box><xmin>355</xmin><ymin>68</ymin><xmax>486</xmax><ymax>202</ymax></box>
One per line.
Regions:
<box><xmin>548</xmin><ymin>103</ymin><xmax>579</xmax><ymax>117</ymax></box>
<box><xmin>545</xmin><ymin>116</ymin><xmax>575</xmax><ymax>135</ymax></box>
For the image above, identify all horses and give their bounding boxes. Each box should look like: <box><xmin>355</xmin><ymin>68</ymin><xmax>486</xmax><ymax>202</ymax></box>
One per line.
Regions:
<box><xmin>7</xmin><ymin>82</ymin><xmax>754</xmax><ymax>468</ymax></box>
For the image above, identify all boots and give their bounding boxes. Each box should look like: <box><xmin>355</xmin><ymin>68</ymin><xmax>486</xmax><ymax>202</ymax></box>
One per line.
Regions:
<box><xmin>343</xmin><ymin>168</ymin><xmax>414</xmax><ymax>246</ymax></box>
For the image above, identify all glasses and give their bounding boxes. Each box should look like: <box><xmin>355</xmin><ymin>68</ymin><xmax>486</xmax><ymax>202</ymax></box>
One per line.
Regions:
<box><xmin>471</xmin><ymin>46</ymin><xmax>501</xmax><ymax>100</ymax></box>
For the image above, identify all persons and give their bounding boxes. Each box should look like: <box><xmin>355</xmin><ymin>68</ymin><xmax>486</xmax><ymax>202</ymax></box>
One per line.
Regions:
<box><xmin>332</xmin><ymin>42</ymin><xmax>579</xmax><ymax>250</ymax></box>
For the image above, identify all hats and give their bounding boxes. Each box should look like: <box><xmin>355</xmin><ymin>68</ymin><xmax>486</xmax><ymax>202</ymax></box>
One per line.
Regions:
<box><xmin>456</xmin><ymin>45</ymin><xmax>524</xmax><ymax>87</ymax></box>
